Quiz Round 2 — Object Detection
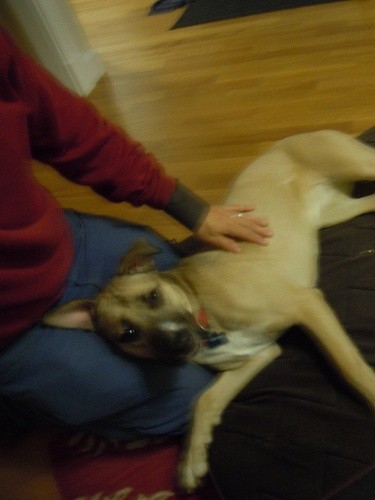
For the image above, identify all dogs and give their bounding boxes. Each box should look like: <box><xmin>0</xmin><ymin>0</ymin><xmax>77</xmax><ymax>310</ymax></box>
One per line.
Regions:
<box><xmin>42</xmin><ymin>129</ymin><xmax>375</xmax><ymax>492</ymax></box>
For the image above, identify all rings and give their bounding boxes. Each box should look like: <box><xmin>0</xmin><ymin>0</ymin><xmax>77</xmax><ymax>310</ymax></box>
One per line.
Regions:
<box><xmin>236</xmin><ymin>212</ymin><xmax>242</xmax><ymax>220</ymax></box>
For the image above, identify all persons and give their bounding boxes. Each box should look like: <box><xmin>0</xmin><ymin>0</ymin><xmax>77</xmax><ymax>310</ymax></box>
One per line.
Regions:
<box><xmin>0</xmin><ymin>26</ymin><xmax>272</xmax><ymax>462</ymax></box>
